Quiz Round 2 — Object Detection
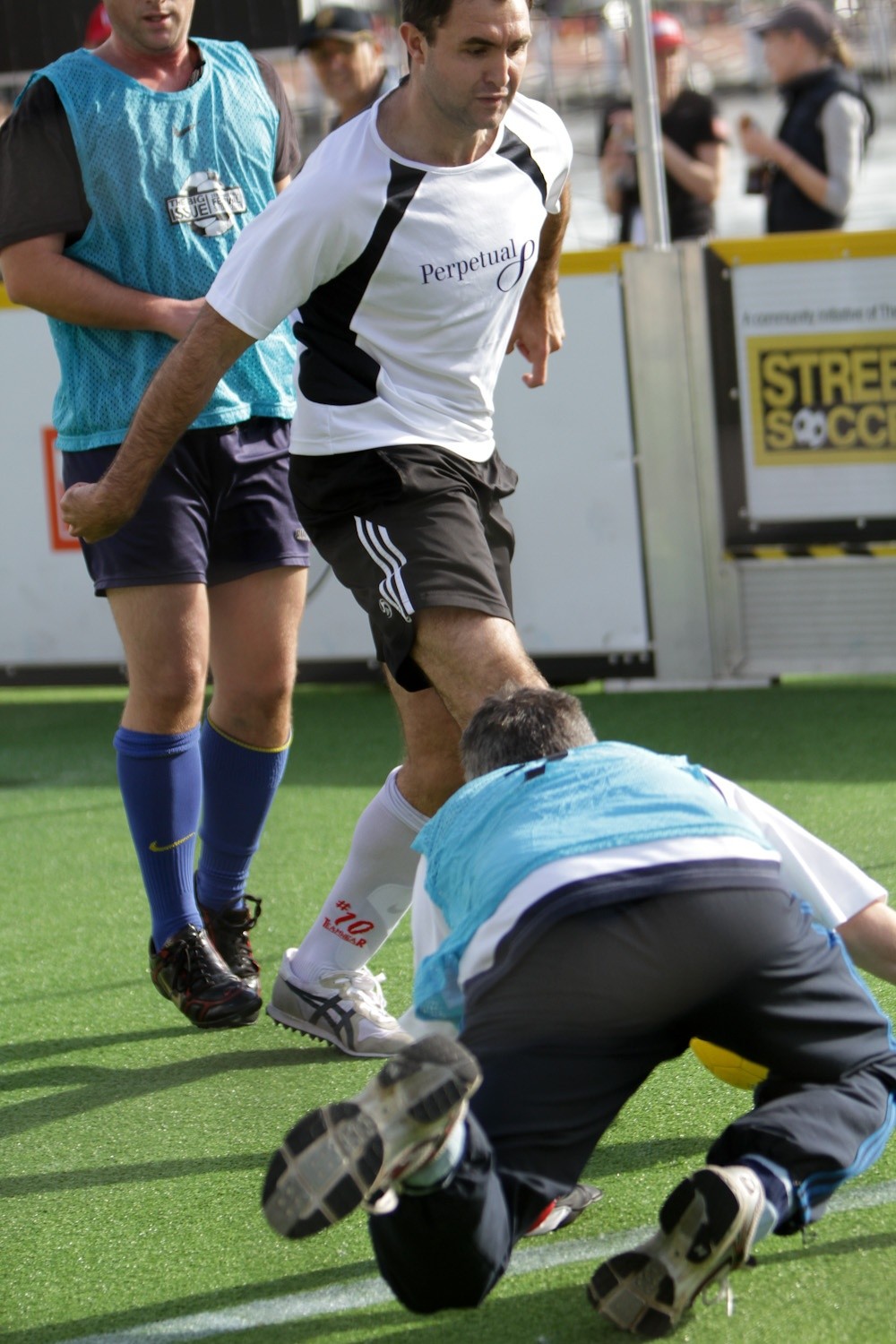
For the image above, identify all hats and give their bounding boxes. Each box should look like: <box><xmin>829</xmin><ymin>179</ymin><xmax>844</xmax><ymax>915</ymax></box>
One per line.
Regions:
<box><xmin>86</xmin><ymin>4</ymin><xmax>112</xmax><ymax>46</ymax></box>
<box><xmin>750</xmin><ymin>3</ymin><xmax>833</xmax><ymax>43</ymax></box>
<box><xmin>648</xmin><ymin>11</ymin><xmax>686</xmax><ymax>50</ymax></box>
<box><xmin>292</xmin><ymin>6</ymin><xmax>375</xmax><ymax>55</ymax></box>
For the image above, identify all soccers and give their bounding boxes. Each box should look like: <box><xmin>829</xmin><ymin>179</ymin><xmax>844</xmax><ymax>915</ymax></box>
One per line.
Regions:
<box><xmin>174</xmin><ymin>166</ymin><xmax>241</xmax><ymax>239</ymax></box>
<box><xmin>689</xmin><ymin>1036</ymin><xmax>770</xmax><ymax>1092</ymax></box>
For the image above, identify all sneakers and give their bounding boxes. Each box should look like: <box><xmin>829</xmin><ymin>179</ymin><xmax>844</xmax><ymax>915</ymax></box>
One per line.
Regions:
<box><xmin>266</xmin><ymin>946</ymin><xmax>415</xmax><ymax>1056</ymax></box>
<box><xmin>260</xmin><ymin>1037</ymin><xmax>482</xmax><ymax>1240</ymax></box>
<box><xmin>147</xmin><ymin>923</ymin><xmax>262</xmax><ymax>1028</ymax></box>
<box><xmin>588</xmin><ymin>1165</ymin><xmax>780</xmax><ymax>1340</ymax></box>
<box><xmin>524</xmin><ymin>1186</ymin><xmax>595</xmax><ymax>1238</ymax></box>
<box><xmin>190</xmin><ymin>868</ymin><xmax>262</xmax><ymax>995</ymax></box>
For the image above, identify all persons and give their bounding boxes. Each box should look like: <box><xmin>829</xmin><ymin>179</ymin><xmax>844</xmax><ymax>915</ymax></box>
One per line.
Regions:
<box><xmin>260</xmin><ymin>678</ymin><xmax>896</xmax><ymax>1338</ymax></box>
<box><xmin>0</xmin><ymin>0</ymin><xmax>303</xmax><ymax>1027</ymax></box>
<box><xmin>57</xmin><ymin>0</ymin><xmax>563</xmax><ymax>1056</ymax></box>
<box><xmin>595</xmin><ymin>17</ymin><xmax>729</xmax><ymax>246</ymax></box>
<box><xmin>290</xmin><ymin>7</ymin><xmax>396</xmax><ymax>138</ymax></box>
<box><xmin>734</xmin><ymin>2</ymin><xmax>879</xmax><ymax>235</ymax></box>
